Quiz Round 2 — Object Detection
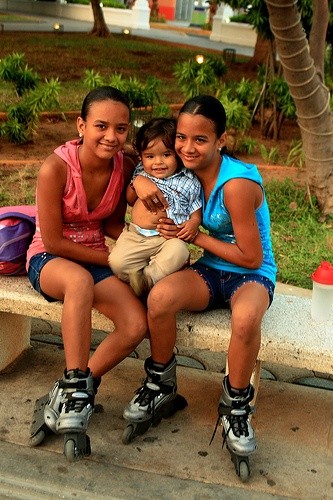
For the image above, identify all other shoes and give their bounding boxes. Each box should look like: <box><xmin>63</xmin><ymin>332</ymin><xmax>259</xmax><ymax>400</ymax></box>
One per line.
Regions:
<box><xmin>129</xmin><ymin>269</ymin><xmax>148</xmax><ymax>297</ymax></box>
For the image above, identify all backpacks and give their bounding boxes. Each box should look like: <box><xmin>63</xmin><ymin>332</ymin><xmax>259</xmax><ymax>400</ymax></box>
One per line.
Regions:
<box><xmin>0</xmin><ymin>202</ymin><xmax>38</xmax><ymax>277</ymax></box>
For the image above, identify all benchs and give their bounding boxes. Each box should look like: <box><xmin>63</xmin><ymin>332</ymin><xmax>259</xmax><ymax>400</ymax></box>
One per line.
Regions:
<box><xmin>1</xmin><ymin>274</ymin><xmax>333</xmax><ymax>382</ymax></box>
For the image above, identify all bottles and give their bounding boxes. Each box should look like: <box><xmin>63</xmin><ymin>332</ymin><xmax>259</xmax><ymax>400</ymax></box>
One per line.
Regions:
<box><xmin>311</xmin><ymin>261</ymin><xmax>333</xmax><ymax>321</ymax></box>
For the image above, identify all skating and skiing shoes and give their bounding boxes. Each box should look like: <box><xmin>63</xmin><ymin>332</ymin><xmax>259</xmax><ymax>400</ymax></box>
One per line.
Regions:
<box><xmin>122</xmin><ymin>353</ymin><xmax>180</xmax><ymax>445</ymax></box>
<box><xmin>56</xmin><ymin>366</ymin><xmax>96</xmax><ymax>461</ymax></box>
<box><xmin>209</xmin><ymin>374</ymin><xmax>258</xmax><ymax>480</ymax></box>
<box><xmin>30</xmin><ymin>377</ymin><xmax>101</xmax><ymax>445</ymax></box>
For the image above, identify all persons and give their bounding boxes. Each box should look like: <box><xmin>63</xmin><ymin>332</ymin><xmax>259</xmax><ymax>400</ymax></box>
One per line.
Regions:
<box><xmin>27</xmin><ymin>86</ymin><xmax>148</xmax><ymax>462</ymax></box>
<box><xmin>107</xmin><ymin>119</ymin><xmax>205</xmax><ymax>294</ymax></box>
<box><xmin>124</xmin><ymin>95</ymin><xmax>276</xmax><ymax>481</ymax></box>
